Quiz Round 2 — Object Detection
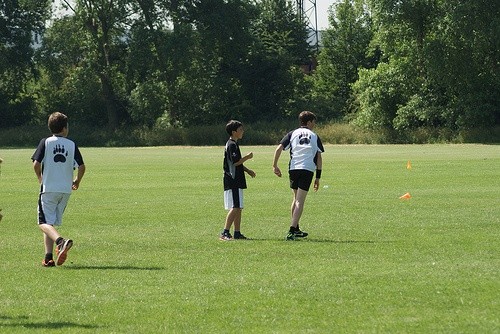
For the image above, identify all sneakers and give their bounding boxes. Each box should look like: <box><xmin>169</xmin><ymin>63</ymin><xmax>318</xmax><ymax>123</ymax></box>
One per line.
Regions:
<box><xmin>220</xmin><ymin>232</ymin><xmax>235</xmax><ymax>240</ymax></box>
<box><xmin>42</xmin><ymin>258</ymin><xmax>55</xmax><ymax>267</ymax></box>
<box><xmin>287</xmin><ymin>233</ymin><xmax>300</xmax><ymax>240</ymax></box>
<box><xmin>54</xmin><ymin>238</ymin><xmax>74</xmax><ymax>267</ymax></box>
<box><xmin>232</xmin><ymin>234</ymin><xmax>246</xmax><ymax>239</ymax></box>
<box><xmin>294</xmin><ymin>228</ymin><xmax>309</xmax><ymax>237</ymax></box>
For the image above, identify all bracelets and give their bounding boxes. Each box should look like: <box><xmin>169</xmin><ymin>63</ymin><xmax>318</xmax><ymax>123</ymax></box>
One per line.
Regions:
<box><xmin>315</xmin><ymin>169</ymin><xmax>322</xmax><ymax>179</ymax></box>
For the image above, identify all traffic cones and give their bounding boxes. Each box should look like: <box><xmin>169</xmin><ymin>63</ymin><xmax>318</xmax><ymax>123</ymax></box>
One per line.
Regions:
<box><xmin>406</xmin><ymin>159</ymin><xmax>412</xmax><ymax>170</ymax></box>
<box><xmin>399</xmin><ymin>192</ymin><xmax>411</xmax><ymax>200</ymax></box>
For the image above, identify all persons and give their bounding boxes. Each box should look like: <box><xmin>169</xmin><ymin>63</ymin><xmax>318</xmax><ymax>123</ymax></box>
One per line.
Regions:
<box><xmin>273</xmin><ymin>111</ymin><xmax>325</xmax><ymax>241</ymax></box>
<box><xmin>220</xmin><ymin>120</ymin><xmax>256</xmax><ymax>241</ymax></box>
<box><xmin>31</xmin><ymin>112</ymin><xmax>86</xmax><ymax>267</ymax></box>
